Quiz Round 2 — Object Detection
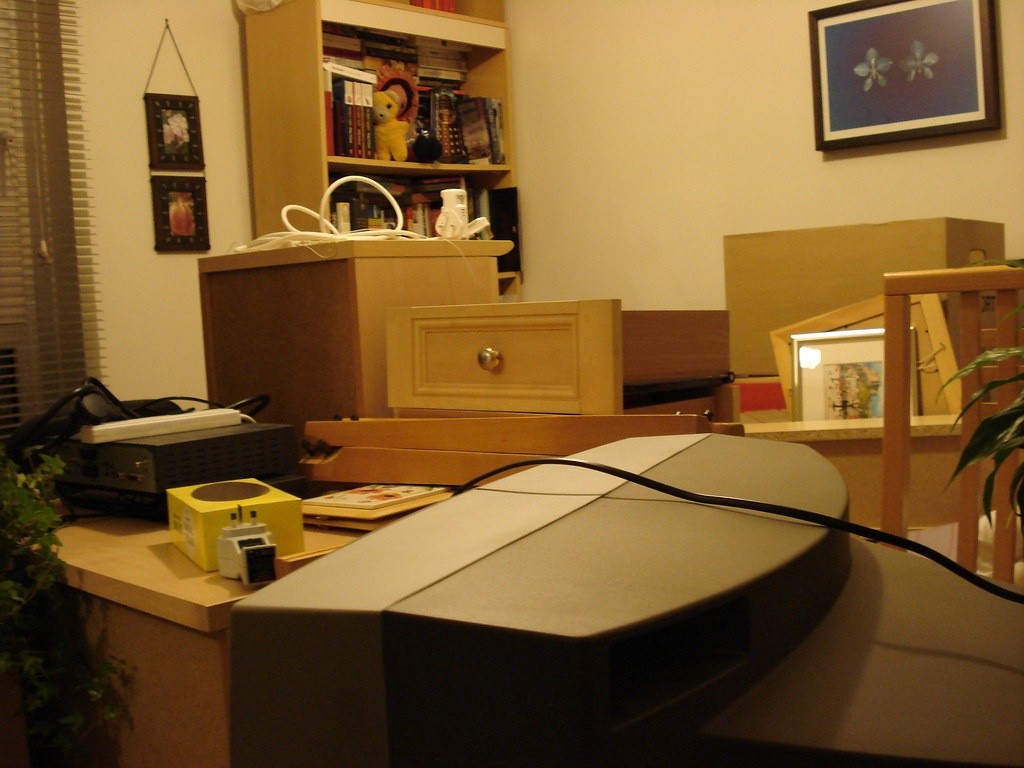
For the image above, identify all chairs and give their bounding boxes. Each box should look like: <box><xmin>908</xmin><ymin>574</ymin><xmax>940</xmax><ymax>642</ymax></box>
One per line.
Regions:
<box><xmin>882</xmin><ymin>266</ymin><xmax>1024</xmax><ymax>584</ymax></box>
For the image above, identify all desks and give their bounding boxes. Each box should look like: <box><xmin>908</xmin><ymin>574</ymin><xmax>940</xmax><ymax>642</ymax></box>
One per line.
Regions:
<box><xmin>1</xmin><ymin>497</ymin><xmax>375</xmax><ymax>768</ymax></box>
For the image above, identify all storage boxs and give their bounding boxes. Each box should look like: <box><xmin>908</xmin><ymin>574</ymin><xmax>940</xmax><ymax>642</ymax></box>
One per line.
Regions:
<box><xmin>165</xmin><ymin>478</ymin><xmax>306</xmax><ymax>574</ymax></box>
<box><xmin>724</xmin><ymin>216</ymin><xmax>1006</xmax><ymax>376</ymax></box>
<box><xmin>727</xmin><ymin>377</ymin><xmax>789</xmax><ymax>425</ymax></box>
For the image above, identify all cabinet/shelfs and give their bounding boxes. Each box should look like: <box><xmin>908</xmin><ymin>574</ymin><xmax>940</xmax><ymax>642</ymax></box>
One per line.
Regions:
<box><xmin>383</xmin><ymin>298</ymin><xmax>728</xmax><ymax>419</ymax></box>
<box><xmin>245</xmin><ymin>0</ymin><xmax>522</xmax><ymax>305</ymax></box>
<box><xmin>198</xmin><ymin>239</ymin><xmax>514</xmax><ymax>462</ymax></box>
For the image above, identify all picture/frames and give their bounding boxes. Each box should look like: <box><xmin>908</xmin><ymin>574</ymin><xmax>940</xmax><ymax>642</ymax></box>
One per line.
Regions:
<box><xmin>769</xmin><ymin>292</ymin><xmax>962</xmax><ymax>423</ymax></box>
<box><xmin>790</xmin><ymin>327</ymin><xmax>919</xmax><ymax>422</ymax></box>
<box><xmin>144</xmin><ymin>94</ymin><xmax>205</xmax><ymax>171</ymax></box>
<box><xmin>808</xmin><ymin>1</ymin><xmax>1003</xmax><ymax>152</ymax></box>
<box><xmin>151</xmin><ymin>175</ymin><xmax>211</xmax><ymax>252</ymax></box>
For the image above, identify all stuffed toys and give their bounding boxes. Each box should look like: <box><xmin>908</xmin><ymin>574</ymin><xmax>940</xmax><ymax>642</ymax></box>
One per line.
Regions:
<box><xmin>374</xmin><ymin>89</ymin><xmax>410</xmax><ymax>162</ymax></box>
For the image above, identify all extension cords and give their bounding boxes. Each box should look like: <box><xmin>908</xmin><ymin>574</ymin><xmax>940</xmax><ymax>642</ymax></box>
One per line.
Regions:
<box><xmin>81</xmin><ymin>408</ymin><xmax>242</xmax><ymax>444</ymax></box>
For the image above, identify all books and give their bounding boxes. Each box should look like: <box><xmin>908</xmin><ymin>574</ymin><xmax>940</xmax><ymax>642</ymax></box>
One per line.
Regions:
<box><xmin>410</xmin><ymin>0</ymin><xmax>457</xmax><ymax>13</ymax></box>
<box><xmin>328</xmin><ymin>172</ymin><xmax>468</xmax><ymax>238</ymax></box>
<box><xmin>321</xmin><ymin>21</ymin><xmax>506</xmax><ymax>165</ymax></box>
<box><xmin>302</xmin><ymin>484</ymin><xmax>455</xmax><ymax>531</ymax></box>
